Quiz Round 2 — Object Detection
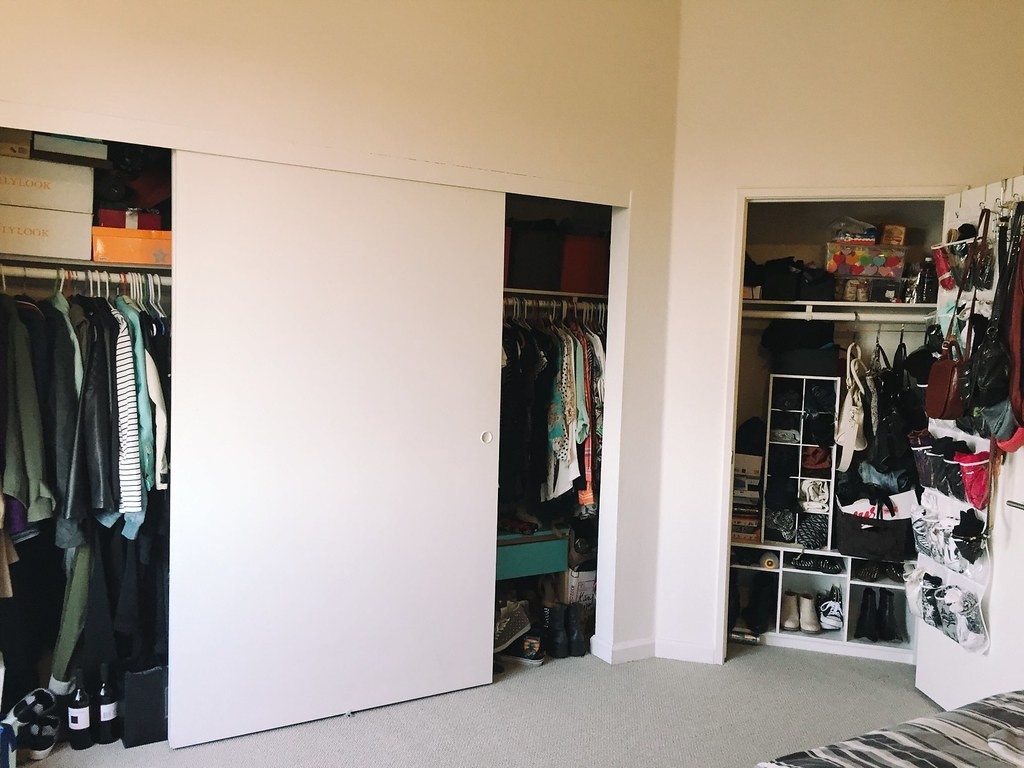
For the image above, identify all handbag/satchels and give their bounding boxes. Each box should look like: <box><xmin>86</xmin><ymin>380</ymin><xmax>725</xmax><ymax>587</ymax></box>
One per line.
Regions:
<box><xmin>927</xmin><ymin>340</ymin><xmax>973</xmax><ymax>420</ymax></box>
<box><xmin>972</xmin><ymin>326</ymin><xmax>1012</xmax><ymax>408</ymax></box>
<box><xmin>836</xmin><ymin>342</ymin><xmax>926</xmax><ymax>451</ymax></box>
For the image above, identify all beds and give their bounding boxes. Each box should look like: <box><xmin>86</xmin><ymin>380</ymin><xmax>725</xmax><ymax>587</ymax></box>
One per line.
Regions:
<box><xmin>752</xmin><ymin>690</ymin><xmax>1024</xmax><ymax>768</ymax></box>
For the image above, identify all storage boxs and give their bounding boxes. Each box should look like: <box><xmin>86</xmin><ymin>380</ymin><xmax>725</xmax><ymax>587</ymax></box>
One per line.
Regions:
<box><xmin>560</xmin><ymin>233</ymin><xmax>610</xmax><ymax>296</ymax></box>
<box><xmin>0</xmin><ymin>156</ymin><xmax>94</xmax><ymax>215</ymax></box>
<box><xmin>92</xmin><ymin>226</ymin><xmax>172</xmax><ymax>266</ymax></box>
<box><xmin>30</xmin><ymin>133</ymin><xmax>109</xmax><ymax>168</ymax></box>
<box><xmin>825</xmin><ymin>242</ymin><xmax>910</xmax><ymax>279</ymax></box>
<box><xmin>831</xmin><ymin>228</ymin><xmax>877</xmax><ymax>245</ymax></box>
<box><xmin>0</xmin><ymin>204</ymin><xmax>93</xmax><ymax>261</ymax></box>
<box><xmin>0</xmin><ymin>127</ymin><xmax>32</xmax><ymax>159</ymax></box>
<box><xmin>496</xmin><ymin>528</ymin><xmax>571</xmax><ymax>579</ymax></box>
<box><xmin>98</xmin><ymin>208</ymin><xmax>162</xmax><ymax>232</ymax></box>
<box><xmin>881</xmin><ymin>223</ymin><xmax>907</xmax><ymax>245</ymax></box>
<box><xmin>835</xmin><ymin>272</ymin><xmax>919</xmax><ymax>304</ymax></box>
<box><xmin>554</xmin><ymin>565</ymin><xmax>597</xmax><ymax>605</ymax></box>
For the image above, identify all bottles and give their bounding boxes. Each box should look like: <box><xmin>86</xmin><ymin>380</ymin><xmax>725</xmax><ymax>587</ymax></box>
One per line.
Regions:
<box><xmin>918</xmin><ymin>257</ymin><xmax>938</xmax><ymax>303</ymax></box>
<box><xmin>65</xmin><ymin>668</ymin><xmax>95</xmax><ymax>750</ymax></box>
<box><xmin>92</xmin><ymin>662</ymin><xmax>120</xmax><ymax>744</ymax></box>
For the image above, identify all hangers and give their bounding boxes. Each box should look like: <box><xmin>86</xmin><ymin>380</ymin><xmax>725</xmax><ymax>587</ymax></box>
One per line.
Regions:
<box><xmin>502</xmin><ymin>297</ymin><xmax>608</xmax><ymax>340</ymax></box>
<box><xmin>0</xmin><ymin>264</ymin><xmax>171</xmax><ymax>336</ymax></box>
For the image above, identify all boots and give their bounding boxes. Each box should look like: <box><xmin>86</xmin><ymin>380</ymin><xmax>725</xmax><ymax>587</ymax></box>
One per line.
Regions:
<box><xmin>781</xmin><ymin>585</ymin><xmax>821</xmax><ymax>634</ymax></box>
<box><xmin>728</xmin><ymin>568</ymin><xmax>777</xmax><ymax>634</ymax></box>
<box><xmin>854</xmin><ymin>587</ymin><xmax>903</xmax><ymax>642</ymax></box>
<box><xmin>536</xmin><ymin>601</ymin><xmax>588</xmax><ymax>658</ymax></box>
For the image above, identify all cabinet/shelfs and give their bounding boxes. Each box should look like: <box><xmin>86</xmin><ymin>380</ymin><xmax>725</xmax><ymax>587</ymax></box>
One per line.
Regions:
<box><xmin>726</xmin><ymin>297</ymin><xmax>940</xmax><ymax>666</ymax></box>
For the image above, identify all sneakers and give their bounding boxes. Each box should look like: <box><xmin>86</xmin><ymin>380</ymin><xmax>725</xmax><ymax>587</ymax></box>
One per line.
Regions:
<box><xmin>494</xmin><ymin>600</ymin><xmax>531</xmax><ymax>654</ymax></box>
<box><xmin>498</xmin><ymin>636</ymin><xmax>546</xmax><ymax>667</ymax></box>
<box><xmin>6</xmin><ymin>688</ymin><xmax>60</xmax><ymax>760</ymax></box>
<box><xmin>815</xmin><ymin>584</ymin><xmax>844</xmax><ymax>631</ymax></box>
